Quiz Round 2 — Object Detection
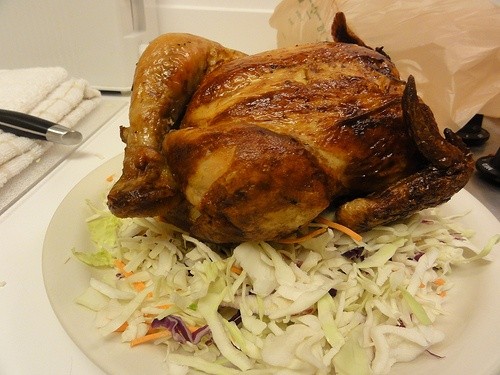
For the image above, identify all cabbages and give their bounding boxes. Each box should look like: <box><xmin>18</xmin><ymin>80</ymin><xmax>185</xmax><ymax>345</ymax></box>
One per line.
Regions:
<box><xmin>69</xmin><ymin>201</ymin><xmax>500</xmax><ymax>375</ymax></box>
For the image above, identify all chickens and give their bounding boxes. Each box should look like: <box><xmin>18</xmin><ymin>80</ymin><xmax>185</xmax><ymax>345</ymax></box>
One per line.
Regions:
<box><xmin>107</xmin><ymin>12</ymin><xmax>473</xmax><ymax>247</ymax></box>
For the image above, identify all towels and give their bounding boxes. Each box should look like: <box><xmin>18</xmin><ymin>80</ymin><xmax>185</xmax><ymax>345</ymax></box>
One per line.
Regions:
<box><xmin>1</xmin><ymin>67</ymin><xmax>102</xmax><ymax>189</ymax></box>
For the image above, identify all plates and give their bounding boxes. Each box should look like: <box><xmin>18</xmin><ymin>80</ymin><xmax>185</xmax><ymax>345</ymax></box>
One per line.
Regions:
<box><xmin>43</xmin><ymin>151</ymin><xmax>500</xmax><ymax>375</ymax></box>
